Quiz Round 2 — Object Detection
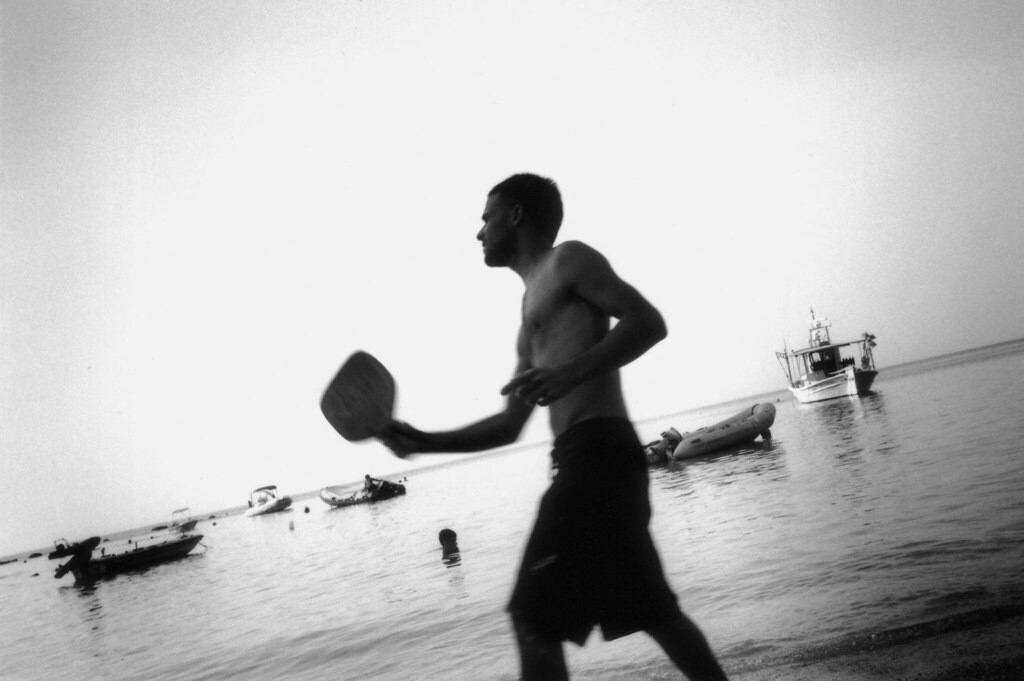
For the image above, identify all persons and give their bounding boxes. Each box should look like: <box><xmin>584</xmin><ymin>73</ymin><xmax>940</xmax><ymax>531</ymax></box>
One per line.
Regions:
<box><xmin>365</xmin><ymin>474</ymin><xmax>383</xmax><ymax>494</ymax></box>
<box><xmin>389</xmin><ymin>173</ymin><xmax>726</xmax><ymax>680</ymax></box>
<box><xmin>437</xmin><ymin>528</ymin><xmax>460</xmax><ymax>558</ymax></box>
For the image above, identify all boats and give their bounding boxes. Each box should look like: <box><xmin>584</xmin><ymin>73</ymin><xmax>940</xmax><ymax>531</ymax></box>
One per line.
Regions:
<box><xmin>55</xmin><ymin>535</ymin><xmax>204</xmax><ymax>581</ymax></box>
<box><xmin>246</xmin><ymin>484</ymin><xmax>292</xmax><ymax>518</ymax></box>
<box><xmin>775</xmin><ymin>307</ymin><xmax>880</xmax><ymax>404</ymax></box>
<box><xmin>318</xmin><ymin>480</ymin><xmax>406</xmax><ymax>506</ymax></box>
<box><xmin>48</xmin><ymin>535</ymin><xmax>100</xmax><ymax>558</ymax></box>
<box><xmin>168</xmin><ymin>506</ymin><xmax>198</xmax><ymax>534</ymax></box>
<box><xmin>640</xmin><ymin>403</ymin><xmax>776</xmax><ymax>462</ymax></box>
